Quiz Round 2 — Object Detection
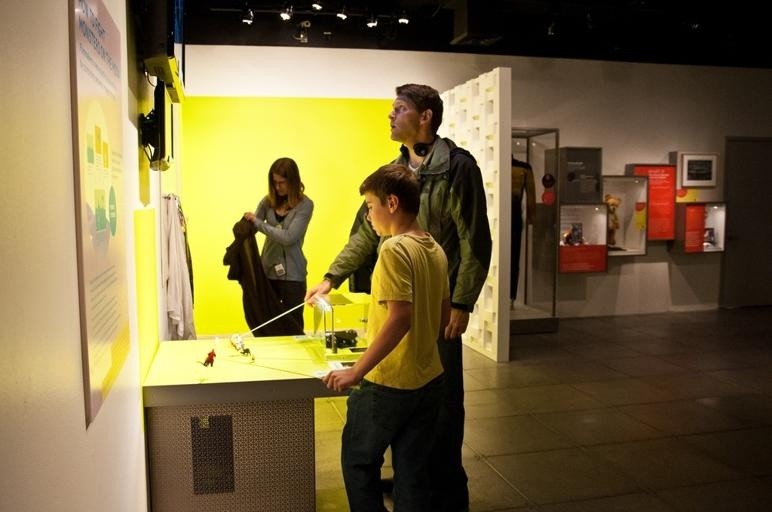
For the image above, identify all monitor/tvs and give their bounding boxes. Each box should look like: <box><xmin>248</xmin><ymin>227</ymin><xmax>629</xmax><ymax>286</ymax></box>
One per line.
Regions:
<box><xmin>141</xmin><ymin>76</ymin><xmax>174</xmax><ymax>172</ymax></box>
<box><xmin>142</xmin><ymin>0</ymin><xmax>186</xmax><ymax>104</ymax></box>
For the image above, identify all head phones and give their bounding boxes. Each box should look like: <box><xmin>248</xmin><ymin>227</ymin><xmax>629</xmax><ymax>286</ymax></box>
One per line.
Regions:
<box><xmin>399</xmin><ymin>135</ymin><xmax>439</xmax><ymax>159</ymax></box>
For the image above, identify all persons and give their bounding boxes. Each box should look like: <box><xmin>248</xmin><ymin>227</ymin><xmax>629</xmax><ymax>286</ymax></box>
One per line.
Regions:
<box><xmin>511</xmin><ymin>152</ymin><xmax>537</xmax><ymax>310</ymax></box>
<box><xmin>304</xmin><ymin>84</ymin><xmax>495</xmax><ymax>511</ymax></box>
<box><xmin>243</xmin><ymin>156</ymin><xmax>315</xmax><ymax>335</ymax></box>
<box><xmin>316</xmin><ymin>163</ymin><xmax>455</xmax><ymax>511</ymax></box>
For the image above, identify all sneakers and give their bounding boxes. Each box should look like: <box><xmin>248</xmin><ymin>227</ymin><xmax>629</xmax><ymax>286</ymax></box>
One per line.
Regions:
<box><xmin>381</xmin><ymin>476</ymin><xmax>395</xmax><ymax>493</ymax></box>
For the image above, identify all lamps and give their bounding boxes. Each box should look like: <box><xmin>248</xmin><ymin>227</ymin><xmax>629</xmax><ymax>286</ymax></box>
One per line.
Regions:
<box><xmin>279</xmin><ymin>5</ymin><xmax>293</xmax><ymax>19</ymax></box>
<box><xmin>242</xmin><ymin>8</ymin><xmax>254</xmax><ymax>23</ymax></box>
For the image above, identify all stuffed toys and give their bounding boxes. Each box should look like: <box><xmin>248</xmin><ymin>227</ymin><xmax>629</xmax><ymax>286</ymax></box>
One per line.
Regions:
<box><xmin>604</xmin><ymin>194</ymin><xmax>623</xmax><ymax>245</ymax></box>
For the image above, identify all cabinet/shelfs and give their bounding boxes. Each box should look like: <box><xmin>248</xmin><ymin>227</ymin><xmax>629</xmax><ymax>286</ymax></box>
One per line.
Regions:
<box><xmin>534</xmin><ymin>148</ymin><xmax>726</xmax><ymax>274</ymax></box>
